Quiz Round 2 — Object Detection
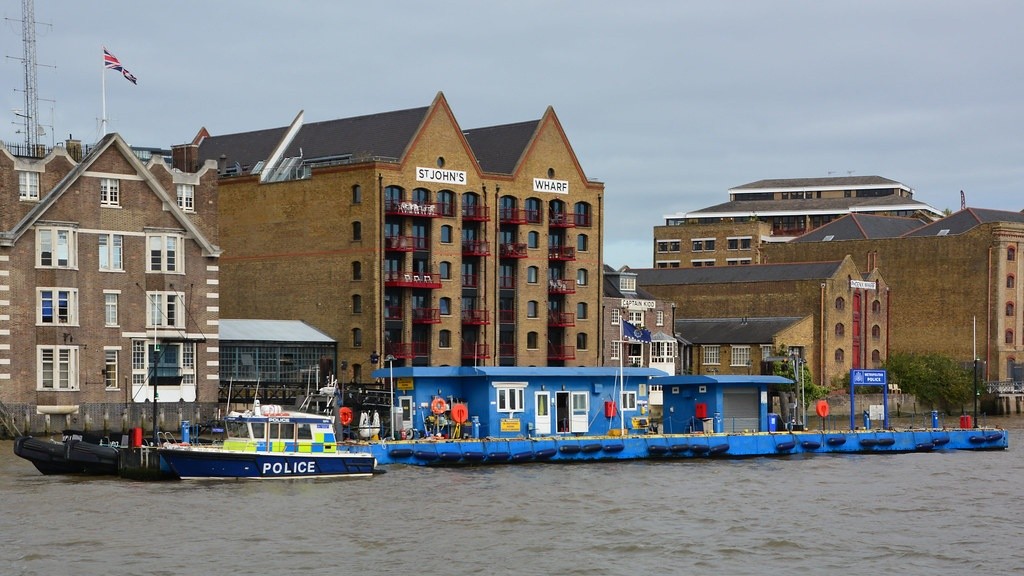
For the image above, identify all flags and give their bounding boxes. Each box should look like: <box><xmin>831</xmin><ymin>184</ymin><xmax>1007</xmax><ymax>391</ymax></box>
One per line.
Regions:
<box><xmin>104</xmin><ymin>46</ymin><xmax>137</xmax><ymax>85</ymax></box>
<box><xmin>622</xmin><ymin>320</ymin><xmax>652</xmax><ymax>344</ymax></box>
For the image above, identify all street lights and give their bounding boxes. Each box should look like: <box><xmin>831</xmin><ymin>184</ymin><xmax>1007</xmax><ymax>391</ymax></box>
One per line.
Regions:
<box><xmin>799</xmin><ymin>358</ymin><xmax>807</xmax><ymax>428</ymax></box>
<box><xmin>351</xmin><ymin>350</ymin><xmax>380</xmax><ymax>383</ymax></box>
<box><xmin>383</xmin><ymin>353</ymin><xmax>398</xmax><ymax>440</ymax></box>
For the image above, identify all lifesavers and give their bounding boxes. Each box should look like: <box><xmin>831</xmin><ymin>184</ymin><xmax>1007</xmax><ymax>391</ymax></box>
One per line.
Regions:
<box><xmin>339</xmin><ymin>407</ymin><xmax>352</xmax><ymax>426</ymax></box>
<box><xmin>431</xmin><ymin>398</ymin><xmax>446</xmax><ymax>414</ymax></box>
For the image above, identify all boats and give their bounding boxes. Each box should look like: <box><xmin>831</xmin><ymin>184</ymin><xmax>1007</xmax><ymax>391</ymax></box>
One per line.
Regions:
<box><xmin>335</xmin><ymin>366</ymin><xmax>1010</xmax><ymax>467</ymax></box>
<box><xmin>298</xmin><ymin>384</ymin><xmax>391</xmax><ymax>451</ymax></box>
<box><xmin>16</xmin><ymin>435</ymin><xmax>101</xmax><ymax>477</ymax></box>
<box><xmin>63</xmin><ymin>430</ymin><xmax>223</xmax><ymax>473</ymax></box>
<box><xmin>157</xmin><ymin>405</ymin><xmax>378</xmax><ymax>480</ymax></box>
